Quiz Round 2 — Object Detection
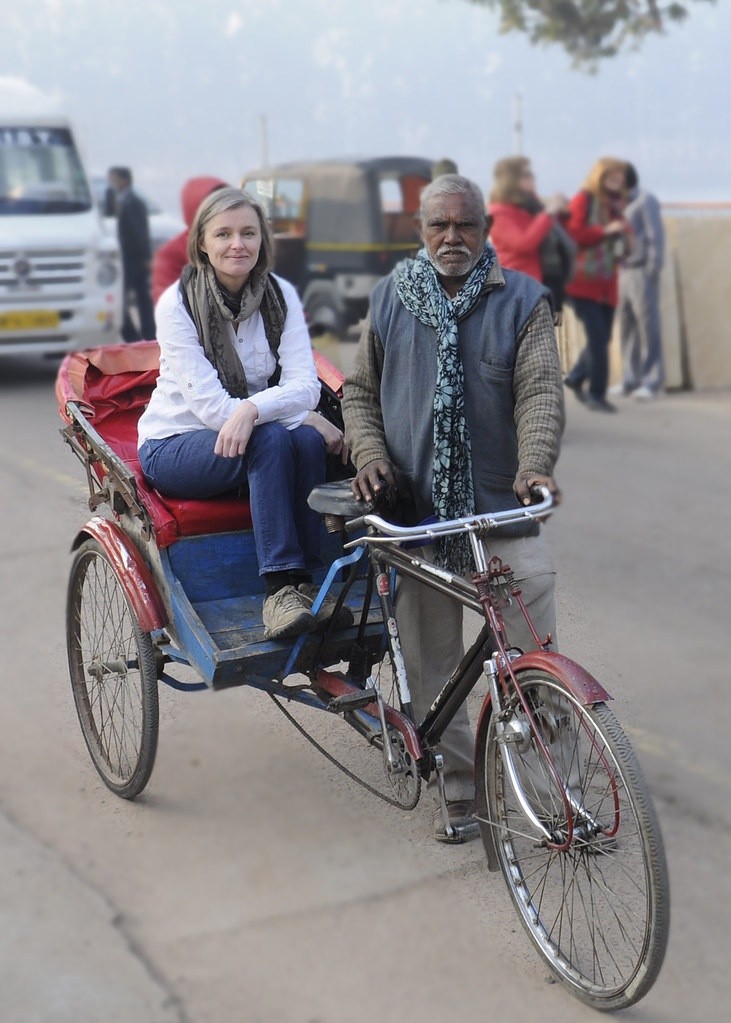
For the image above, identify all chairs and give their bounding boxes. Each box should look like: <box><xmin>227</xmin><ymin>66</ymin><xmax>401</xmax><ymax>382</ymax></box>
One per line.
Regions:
<box><xmin>385</xmin><ymin>212</ymin><xmax>417</xmax><ymax>242</ymax></box>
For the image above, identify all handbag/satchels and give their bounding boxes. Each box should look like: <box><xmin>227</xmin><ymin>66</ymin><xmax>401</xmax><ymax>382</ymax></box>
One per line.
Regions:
<box><xmin>530</xmin><ymin>203</ymin><xmax>576</xmax><ymax>282</ymax></box>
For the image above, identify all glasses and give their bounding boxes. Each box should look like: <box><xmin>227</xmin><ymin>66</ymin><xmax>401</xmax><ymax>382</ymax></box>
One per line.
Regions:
<box><xmin>521</xmin><ymin>171</ymin><xmax>533</xmax><ymax>179</ymax></box>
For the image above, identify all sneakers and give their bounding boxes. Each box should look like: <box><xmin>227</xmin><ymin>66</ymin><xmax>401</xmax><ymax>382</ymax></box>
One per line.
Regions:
<box><xmin>298</xmin><ymin>582</ymin><xmax>354</xmax><ymax>629</ymax></box>
<box><xmin>262</xmin><ymin>585</ymin><xmax>316</xmax><ymax>639</ymax></box>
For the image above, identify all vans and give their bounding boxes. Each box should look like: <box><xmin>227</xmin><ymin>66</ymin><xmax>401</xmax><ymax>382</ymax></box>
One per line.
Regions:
<box><xmin>233</xmin><ymin>155</ymin><xmax>438</xmax><ymax>342</ymax></box>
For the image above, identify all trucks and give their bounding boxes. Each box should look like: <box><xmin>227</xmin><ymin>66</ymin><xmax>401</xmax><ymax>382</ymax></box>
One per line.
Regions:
<box><xmin>0</xmin><ymin>72</ymin><xmax>123</xmax><ymax>365</ymax></box>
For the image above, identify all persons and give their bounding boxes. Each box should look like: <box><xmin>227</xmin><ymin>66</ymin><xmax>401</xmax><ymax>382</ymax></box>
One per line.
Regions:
<box><xmin>339</xmin><ymin>174</ymin><xmax>588</xmax><ymax>842</ymax></box>
<box><xmin>137</xmin><ymin>186</ymin><xmax>347</xmax><ymax>638</ymax></box>
<box><xmin>101</xmin><ymin>154</ymin><xmax>665</xmax><ymax>415</ymax></box>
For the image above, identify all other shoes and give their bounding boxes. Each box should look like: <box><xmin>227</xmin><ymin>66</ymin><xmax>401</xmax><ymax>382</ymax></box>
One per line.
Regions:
<box><xmin>607</xmin><ymin>384</ymin><xmax>624</xmax><ymax>395</ymax></box>
<box><xmin>631</xmin><ymin>387</ymin><xmax>663</xmax><ymax>400</ymax></box>
<box><xmin>537</xmin><ymin>721</ymin><xmax>583</xmax><ymax>790</ymax></box>
<box><xmin>583</xmin><ymin>392</ymin><xmax>615</xmax><ymax>411</ymax></box>
<box><xmin>565</xmin><ymin>376</ymin><xmax>587</xmax><ymax>402</ymax></box>
<box><xmin>432</xmin><ymin>802</ymin><xmax>479</xmax><ymax>842</ymax></box>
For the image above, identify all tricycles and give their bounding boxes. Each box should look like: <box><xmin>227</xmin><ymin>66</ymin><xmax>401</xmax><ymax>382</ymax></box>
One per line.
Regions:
<box><xmin>55</xmin><ymin>330</ymin><xmax>672</xmax><ymax>1013</ymax></box>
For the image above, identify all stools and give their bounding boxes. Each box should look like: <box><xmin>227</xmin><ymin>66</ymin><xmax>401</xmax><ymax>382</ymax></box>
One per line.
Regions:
<box><xmin>122</xmin><ymin>455</ymin><xmax>252</xmax><ymax>538</ymax></box>
